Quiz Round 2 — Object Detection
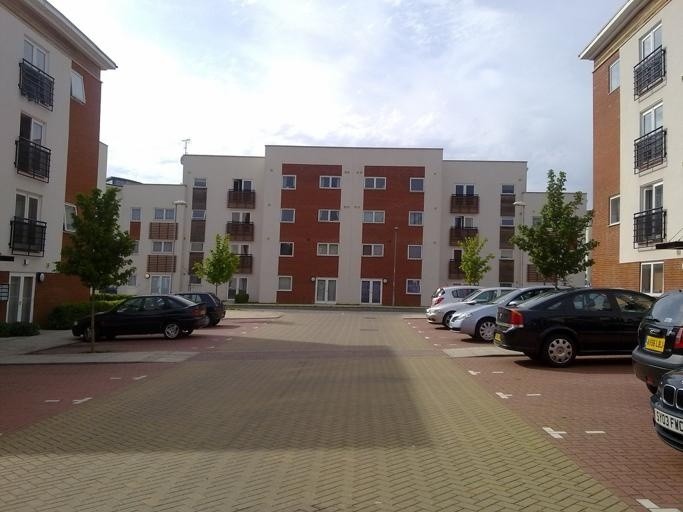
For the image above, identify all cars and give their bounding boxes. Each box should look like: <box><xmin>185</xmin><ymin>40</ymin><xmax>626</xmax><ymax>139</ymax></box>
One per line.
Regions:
<box><xmin>72</xmin><ymin>292</ymin><xmax>226</xmax><ymax>343</ymax></box>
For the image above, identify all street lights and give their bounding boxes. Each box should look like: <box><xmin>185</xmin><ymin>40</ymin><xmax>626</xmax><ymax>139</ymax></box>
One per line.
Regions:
<box><xmin>513</xmin><ymin>201</ymin><xmax>528</xmax><ymax>288</ymax></box>
<box><xmin>169</xmin><ymin>200</ymin><xmax>187</xmax><ymax>294</ymax></box>
<box><xmin>388</xmin><ymin>226</ymin><xmax>400</xmax><ymax>306</ymax></box>
